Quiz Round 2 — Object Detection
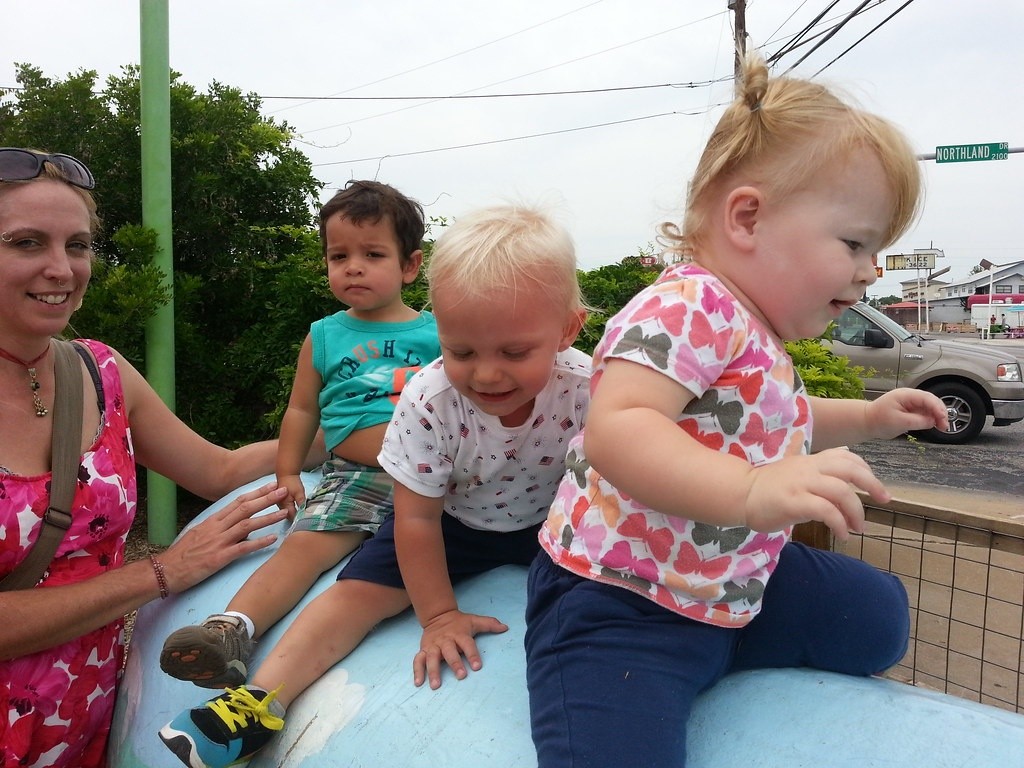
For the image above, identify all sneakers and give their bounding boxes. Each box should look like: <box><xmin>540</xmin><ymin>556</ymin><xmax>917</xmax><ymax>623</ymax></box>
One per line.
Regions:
<box><xmin>158</xmin><ymin>682</ymin><xmax>286</xmax><ymax>768</ymax></box>
<box><xmin>160</xmin><ymin>614</ymin><xmax>258</xmax><ymax>689</ymax></box>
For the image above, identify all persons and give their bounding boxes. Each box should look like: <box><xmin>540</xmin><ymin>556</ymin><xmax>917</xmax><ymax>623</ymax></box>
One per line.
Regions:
<box><xmin>1</xmin><ymin>151</ymin><xmax>331</xmax><ymax>768</ymax></box>
<box><xmin>525</xmin><ymin>59</ymin><xmax>949</xmax><ymax>768</ymax></box>
<box><xmin>161</xmin><ymin>180</ymin><xmax>442</xmax><ymax>690</ymax></box>
<box><xmin>157</xmin><ymin>205</ymin><xmax>593</xmax><ymax>768</ymax></box>
<box><xmin>991</xmin><ymin>315</ymin><xmax>996</xmax><ymax>324</ymax></box>
<box><xmin>1002</xmin><ymin>314</ymin><xmax>1006</xmax><ymax>327</ymax></box>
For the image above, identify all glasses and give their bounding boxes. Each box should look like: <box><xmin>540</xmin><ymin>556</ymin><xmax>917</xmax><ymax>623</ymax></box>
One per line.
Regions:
<box><xmin>0</xmin><ymin>147</ymin><xmax>96</xmax><ymax>190</ymax></box>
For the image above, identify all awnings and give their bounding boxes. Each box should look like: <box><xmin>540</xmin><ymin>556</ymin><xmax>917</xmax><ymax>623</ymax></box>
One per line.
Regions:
<box><xmin>967</xmin><ymin>294</ymin><xmax>1024</xmax><ymax>308</ymax></box>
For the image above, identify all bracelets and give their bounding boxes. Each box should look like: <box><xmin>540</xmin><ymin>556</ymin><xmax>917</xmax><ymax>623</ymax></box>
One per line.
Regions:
<box><xmin>151</xmin><ymin>554</ymin><xmax>169</xmax><ymax>599</ymax></box>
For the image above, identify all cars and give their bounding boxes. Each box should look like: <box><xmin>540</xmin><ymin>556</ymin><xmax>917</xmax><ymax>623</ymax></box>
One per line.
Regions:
<box><xmin>820</xmin><ymin>300</ymin><xmax>1024</xmax><ymax>445</ymax></box>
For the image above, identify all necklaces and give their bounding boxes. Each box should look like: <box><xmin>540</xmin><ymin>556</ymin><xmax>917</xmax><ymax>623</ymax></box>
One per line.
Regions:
<box><xmin>0</xmin><ymin>340</ymin><xmax>51</xmax><ymax>416</ymax></box>
